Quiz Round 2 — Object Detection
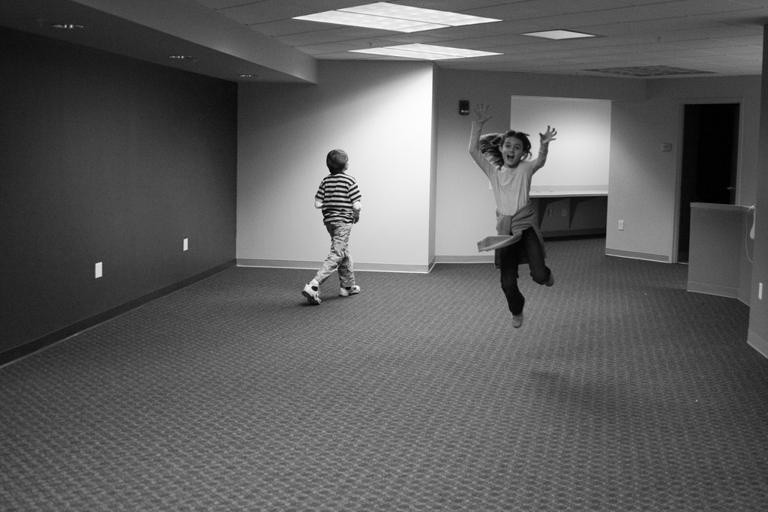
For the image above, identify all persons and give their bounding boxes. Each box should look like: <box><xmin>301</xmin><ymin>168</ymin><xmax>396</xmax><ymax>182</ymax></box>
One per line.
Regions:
<box><xmin>470</xmin><ymin>103</ymin><xmax>556</xmax><ymax>328</ymax></box>
<box><xmin>300</xmin><ymin>148</ymin><xmax>364</xmax><ymax>305</ymax></box>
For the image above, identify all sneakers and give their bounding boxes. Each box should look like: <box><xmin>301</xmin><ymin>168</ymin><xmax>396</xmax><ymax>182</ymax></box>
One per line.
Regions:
<box><xmin>340</xmin><ymin>284</ymin><xmax>360</xmax><ymax>296</ymax></box>
<box><xmin>301</xmin><ymin>284</ymin><xmax>320</xmax><ymax>305</ymax></box>
<box><xmin>545</xmin><ymin>272</ymin><xmax>554</xmax><ymax>286</ymax></box>
<box><xmin>512</xmin><ymin>312</ymin><xmax>522</xmax><ymax>327</ymax></box>
